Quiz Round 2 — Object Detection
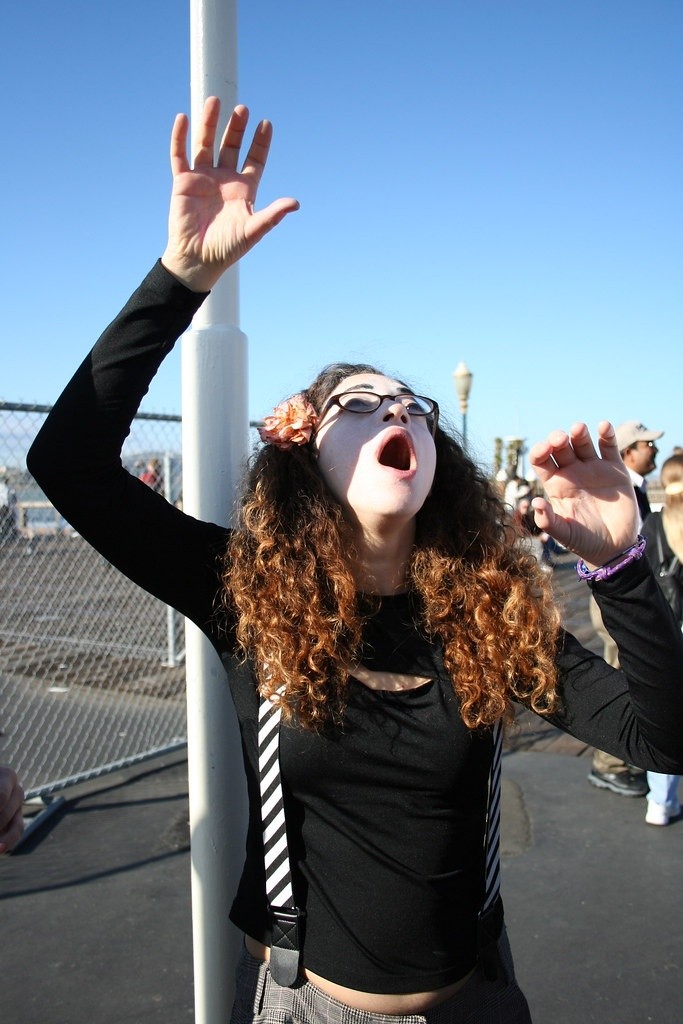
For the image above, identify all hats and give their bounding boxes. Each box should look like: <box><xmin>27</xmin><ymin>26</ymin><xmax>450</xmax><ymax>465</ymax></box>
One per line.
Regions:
<box><xmin>615</xmin><ymin>420</ymin><xmax>665</xmax><ymax>451</ymax></box>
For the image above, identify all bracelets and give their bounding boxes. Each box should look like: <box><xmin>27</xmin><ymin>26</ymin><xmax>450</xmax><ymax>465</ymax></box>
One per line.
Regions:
<box><xmin>577</xmin><ymin>536</ymin><xmax>646</xmax><ymax>582</ymax></box>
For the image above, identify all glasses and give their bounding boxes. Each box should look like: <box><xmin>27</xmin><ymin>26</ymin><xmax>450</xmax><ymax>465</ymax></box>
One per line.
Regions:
<box><xmin>312</xmin><ymin>392</ymin><xmax>439</xmax><ymax>439</ymax></box>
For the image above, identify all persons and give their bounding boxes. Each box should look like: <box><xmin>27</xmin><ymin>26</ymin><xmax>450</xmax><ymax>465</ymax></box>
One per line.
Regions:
<box><xmin>589</xmin><ymin>424</ymin><xmax>683</xmax><ymax>826</ymax></box>
<box><xmin>27</xmin><ymin>97</ymin><xmax>683</xmax><ymax>1024</ymax></box>
<box><xmin>128</xmin><ymin>458</ymin><xmax>183</xmax><ymax>512</ymax></box>
<box><xmin>503</xmin><ymin>471</ymin><xmax>570</xmax><ymax>572</ymax></box>
<box><xmin>0</xmin><ymin>472</ymin><xmax>17</xmax><ymax>548</ymax></box>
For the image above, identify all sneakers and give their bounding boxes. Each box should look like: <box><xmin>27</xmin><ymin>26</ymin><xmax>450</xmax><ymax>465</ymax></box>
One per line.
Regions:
<box><xmin>587</xmin><ymin>768</ymin><xmax>648</xmax><ymax>796</ymax></box>
<box><xmin>646</xmin><ymin>801</ymin><xmax>681</xmax><ymax>825</ymax></box>
